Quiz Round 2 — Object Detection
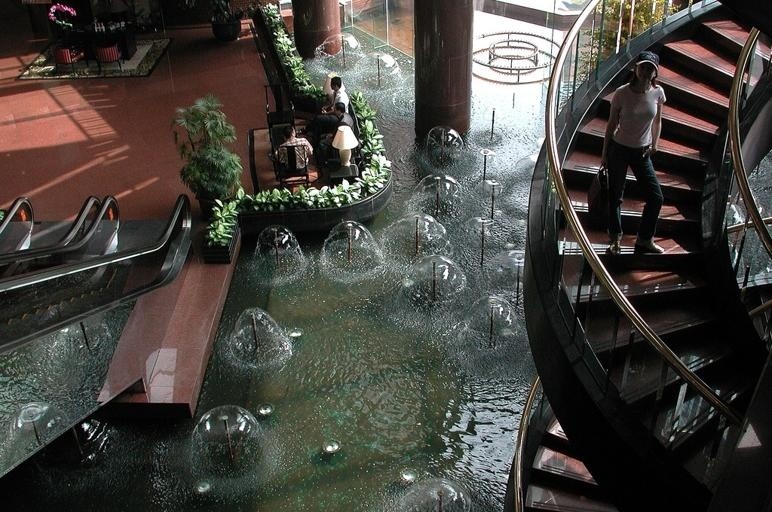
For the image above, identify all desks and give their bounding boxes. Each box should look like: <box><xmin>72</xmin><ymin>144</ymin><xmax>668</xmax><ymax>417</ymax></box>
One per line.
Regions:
<box><xmin>328</xmin><ymin>158</ymin><xmax>359</xmax><ymax>178</ymax></box>
<box><xmin>61</xmin><ymin>17</ymin><xmax>138</xmax><ymax>60</ymax></box>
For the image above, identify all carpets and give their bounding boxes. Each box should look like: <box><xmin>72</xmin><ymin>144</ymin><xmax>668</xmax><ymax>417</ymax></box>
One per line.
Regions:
<box><xmin>248</xmin><ymin>125</ymin><xmax>342</xmax><ymax>195</ymax></box>
<box><xmin>15</xmin><ymin>37</ymin><xmax>172</xmax><ymax>79</ymax></box>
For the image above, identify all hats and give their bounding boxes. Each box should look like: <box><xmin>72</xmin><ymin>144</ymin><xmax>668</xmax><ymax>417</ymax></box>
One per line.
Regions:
<box><xmin>636</xmin><ymin>52</ymin><xmax>659</xmax><ymax>71</ymax></box>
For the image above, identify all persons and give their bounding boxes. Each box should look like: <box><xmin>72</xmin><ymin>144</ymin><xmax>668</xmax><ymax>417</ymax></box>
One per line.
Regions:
<box><xmin>300</xmin><ymin>77</ymin><xmax>358</xmax><ymax>171</ymax></box>
<box><xmin>269</xmin><ymin>128</ymin><xmax>312</xmax><ymax>176</ymax></box>
<box><xmin>600</xmin><ymin>52</ymin><xmax>669</xmax><ymax>255</ymax></box>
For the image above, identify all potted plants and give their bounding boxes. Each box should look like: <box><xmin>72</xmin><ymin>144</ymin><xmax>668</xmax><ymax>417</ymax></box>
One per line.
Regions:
<box><xmin>199</xmin><ymin>199</ymin><xmax>240</xmax><ymax>264</ymax></box>
<box><xmin>169</xmin><ymin>92</ymin><xmax>243</xmax><ymax>221</ymax></box>
<box><xmin>208</xmin><ymin>0</ymin><xmax>244</xmax><ymax>41</ymax></box>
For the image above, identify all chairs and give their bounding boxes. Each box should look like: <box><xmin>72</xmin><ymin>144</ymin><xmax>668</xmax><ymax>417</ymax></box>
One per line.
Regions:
<box><xmin>263</xmin><ymin>83</ymin><xmax>309</xmax><ymax>189</ymax></box>
<box><xmin>54</xmin><ymin>43</ymin><xmax>124</xmax><ymax>76</ymax></box>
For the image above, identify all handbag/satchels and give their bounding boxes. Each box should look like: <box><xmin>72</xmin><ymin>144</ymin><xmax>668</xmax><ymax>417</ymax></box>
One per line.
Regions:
<box><xmin>588</xmin><ymin>163</ymin><xmax>611</xmax><ymax>226</ymax></box>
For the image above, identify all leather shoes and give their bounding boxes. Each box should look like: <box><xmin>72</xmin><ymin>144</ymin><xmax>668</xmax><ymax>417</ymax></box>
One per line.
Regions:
<box><xmin>611</xmin><ymin>240</ymin><xmax>620</xmax><ymax>256</ymax></box>
<box><xmin>635</xmin><ymin>240</ymin><xmax>665</xmax><ymax>252</ymax></box>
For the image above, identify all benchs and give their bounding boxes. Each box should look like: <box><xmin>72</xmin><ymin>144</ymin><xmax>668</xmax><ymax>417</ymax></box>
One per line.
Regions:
<box><xmin>316</xmin><ymin>100</ymin><xmax>360</xmax><ymax>168</ymax></box>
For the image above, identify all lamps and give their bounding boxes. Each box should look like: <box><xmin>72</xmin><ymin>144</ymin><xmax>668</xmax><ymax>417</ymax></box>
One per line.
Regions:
<box><xmin>323</xmin><ymin>72</ymin><xmax>346</xmax><ymax>104</ymax></box>
<box><xmin>332</xmin><ymin>126</ymin><xmax>359</xmax><ymax>167</ymax></box>
